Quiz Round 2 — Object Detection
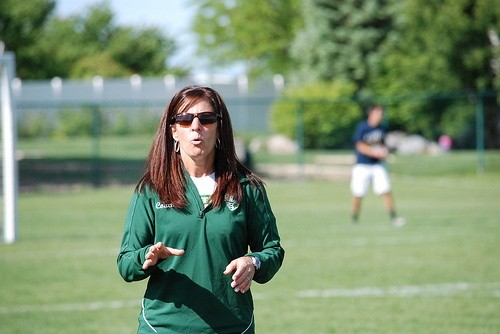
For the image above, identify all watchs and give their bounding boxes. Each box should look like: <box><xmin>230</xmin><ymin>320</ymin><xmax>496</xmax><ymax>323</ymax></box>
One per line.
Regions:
<box><xmin>251</xmin><ymin>256</ymin><xmax>260</xmax><ymax>273</ymax></box>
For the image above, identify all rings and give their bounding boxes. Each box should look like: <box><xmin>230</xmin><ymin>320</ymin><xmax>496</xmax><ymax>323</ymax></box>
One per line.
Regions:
<box><xmin>246</xmin><ymin>277</ymin><xmax>251</xmax><ymax>282</ymax></box>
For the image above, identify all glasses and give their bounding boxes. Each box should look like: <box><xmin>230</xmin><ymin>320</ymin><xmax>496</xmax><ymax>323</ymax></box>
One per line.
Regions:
<box><xmin>168</xmin><ymin>111</ymin><xmax>222</xmax><ymax>127</ymax></box>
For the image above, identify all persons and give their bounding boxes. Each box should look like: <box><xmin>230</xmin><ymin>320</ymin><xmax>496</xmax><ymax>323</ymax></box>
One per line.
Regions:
<box><xmin>347</xmin><ymin>106</ymin><xmax>406</xmax><ymax>228</ymax></box>
<box><xmin>116</xmin><ymin>86</ymin><xmax>284</xmax><ymax>334</ymax></box>
